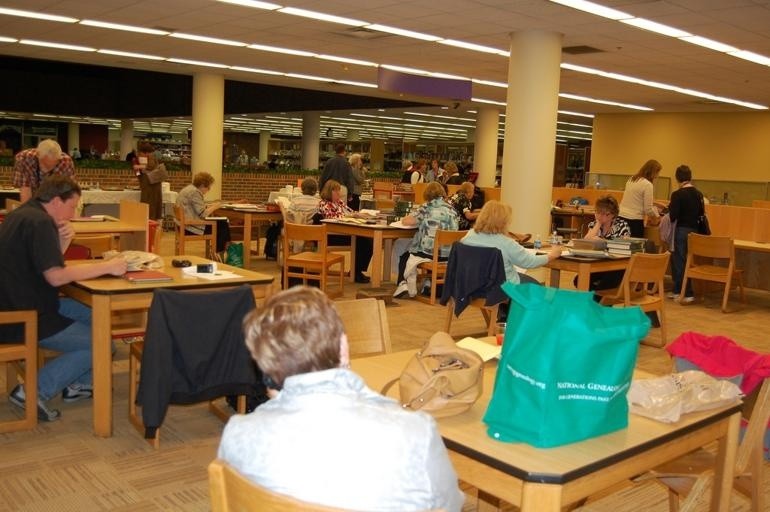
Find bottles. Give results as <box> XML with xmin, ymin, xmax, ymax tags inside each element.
<box><xmin>534</xmin><ymin>234</ymin><xmax>541</xmax><ymax>249</ymax></box>
<box><xmin>724</xmin><ymin>193</ymin><xmax>728</xmax><ymax>205</ymax></box>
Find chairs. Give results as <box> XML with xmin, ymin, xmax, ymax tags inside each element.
<box><xmin>69</xmin><ymin>233</ymin><xmax>119</xmax><ymax>260</ymax></box>
<box><xmin>643</xmin><ymin>331</ymin><xmax>770</xmax><ymax>511</ymax></box>
<box><xmin>599</xmin><ymin>252</ymin><xmax>671</xmax><ymax>346</ymax></box>
<box><xmin>209</xmin><ymin>459</ymin><xmax>342</xmax><ymax>511</ymax></box>
<box><xmin>418</xmin><ymin>229</ymin><xmax>470</xmax><ymax>306</ymax></box>
<box><xmin>334</xmin><ymin>298</ymin><xmax>391</xmax><ymax>358</ymax></box>
<box><xmin>117</xmin><ymin>200</ymin><xmax>149</xmax><ymax>253</ymax></box>
<box><xmin>373</xmin><ymin>182</ymin><xmax>396</xmax><ymax>213</ymax></box>
<box><xmin>678</xmin><ymin>233</ymin><xmax>746</xmax><ymax>313</ymax></box>
<box><xmin>1</xmin><ymin>310</ymin><xmax>37</xmax><ymax>433</ymax></box>
<box><xmin>283</xmin><ymin>222</ymin><xmax>345</xmax><ymax>298</ymax></box>
<box><xmin>173</xmin><ymin>205</ymin><xmax>216</xmax><ymax>263</ymax></box>
<box><xmin>129</xmin><ymin>284</ymin><xmax>253</xmax><ymax>450</ymax></box>
<box><xmin>444</xmin><ymin>241</ymin><xmax>511</xmax><ymax>337</ymax></box>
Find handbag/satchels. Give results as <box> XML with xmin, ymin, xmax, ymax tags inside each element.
<box><xmin>226</xmin><ymin>242</ymin><xmax>244</xmax><ymax>267</ymax></box>
<box><xmin>695</xmin><ymin>215</ymin><xmax>712</xmax><ymax>235</ymax></box>
<box><xmin>379</xmin><ymin>331</ymin><xmax>484</xmax><ymax>417</ymax></box>
<box><xmin>146</xmin><ymin>162</ymin><xmax>168</xmax><ymax>185</ymax></box>
<box><xmin>482</xmin><ymin>282</ymin><xmax>652</xmax><ymax>449</ymax></box>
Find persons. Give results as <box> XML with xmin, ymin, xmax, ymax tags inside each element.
<box><xmin>216</xmin><ymin>285</ymin><xmax>463</xmax><ymax>512</ymax></box>
<box><xmin>73</xmin><ymin>148</ymin><xmax>82</xmax><ymax>161</ymax></box>
<box><xmin>0</xmin><ymin>176</ymin><xmax>115</xmax><ymax>421</ymax></box>
<box><xmin>12</xmin><ymin>138</ymin><xmax>75</xmax><ymax>202</ymax></box>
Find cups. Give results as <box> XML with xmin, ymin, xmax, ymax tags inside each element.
<box><xmin>286</xmin><ymin>185</ymin><xmax>302</xmax><ymax>194</ymax></box>
<box><xmin>386</xmin><ymin>215</ymin><xmax>399</xmax><ymax>225</ymax></box>
<box><xmin>494</xmin><ymin>323</ymin><xmax>507</xmax><ymax>346</ymax></box>
<box><xmin>396</xmin><ymin>201</ymin><xmax>413</xmax><ymax>217</ymax></box>
<box><xmin>197</xmin><ymin>263</ymin><xmax>214</xmax><ymax>273</ymax></box>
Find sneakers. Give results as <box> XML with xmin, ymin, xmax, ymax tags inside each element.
<box><xmin>62</xmin><ymin>385</ymin><xmax>93</xmax><ymax>402</ymax></box>
<box><xmin>9</xmin><ymin>383</ymin><xmax>60</xmax><ymax>422</ymax></box>
<box><xmin>393</xmin><ymin>283</ymin><xmax>410</xmax><ymax>298</ymax></box>
<box><xmin>668</xmin><ymin>291</ymin><xmax>697</xmax><ymax>302</ymax></box>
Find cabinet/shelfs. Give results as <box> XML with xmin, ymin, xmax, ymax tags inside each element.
<box><xmin>383</xmin><ymin>139</ymin><xmax>405</xmax><ymax>169</ymax></box>
<box><xmin>137</xmin><ymin>140</ymin><xmax>191</xmax><ymax>158</ymax></box>
<box><xmin>267</xmin><ymin>138</ymin><xmax>384</xmax><ymax>171</ymax></box>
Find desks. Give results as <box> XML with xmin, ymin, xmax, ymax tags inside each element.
<box><xmin>61</xmin><ymin>255</ymin><xmax>274</xmax><ymax>438</ymax></box>
<box><xmin>80</xmin><ymin>185</ymin><xmax>179</xmax><ymax>205</ymax></box>
<box><xmin>474</xmin><ymin>208</ymin><xmax>481</xmax><ymax>226</ymax></box>
<box><xmin>70</xmin><ymin>219</ymin><xmax>145</xmax><ymax>235</ymax></box>
<box><xmin>270</xmin><ymin>190</ymin><xmax>415</xmax><ymax>206</ymax></box>
<box><xmin>735</xmin><ymin>239</ymin><xmax>769</xmax><ymax>252</ymax></box>
<box><xmin>1</xmin><ymin>186</ymin><xmax>21</xmax><ymax>209</ymax></box>
<box><xmin>208</xmin><ymin>201</ymin><xmax>283</xmax><ymax>272</ymax></box>
<box><xmin>351</xmin><ymin>333</ymin><xmax>744</xmax><ymax>510</ymax></box>
<box><xmin>552</xmin><ymin>204</ymin><xmax>621</xmax><ymax>238</ymax></box>
<box><xmin>524</xmin><ymin>244</ymin><xmax>632</xmax><ymax>293</ymax></box>
<box><xmin>321</xmin><ymin>216</ymin><xmax>420</xmax><ymax>288</ymax></box>
